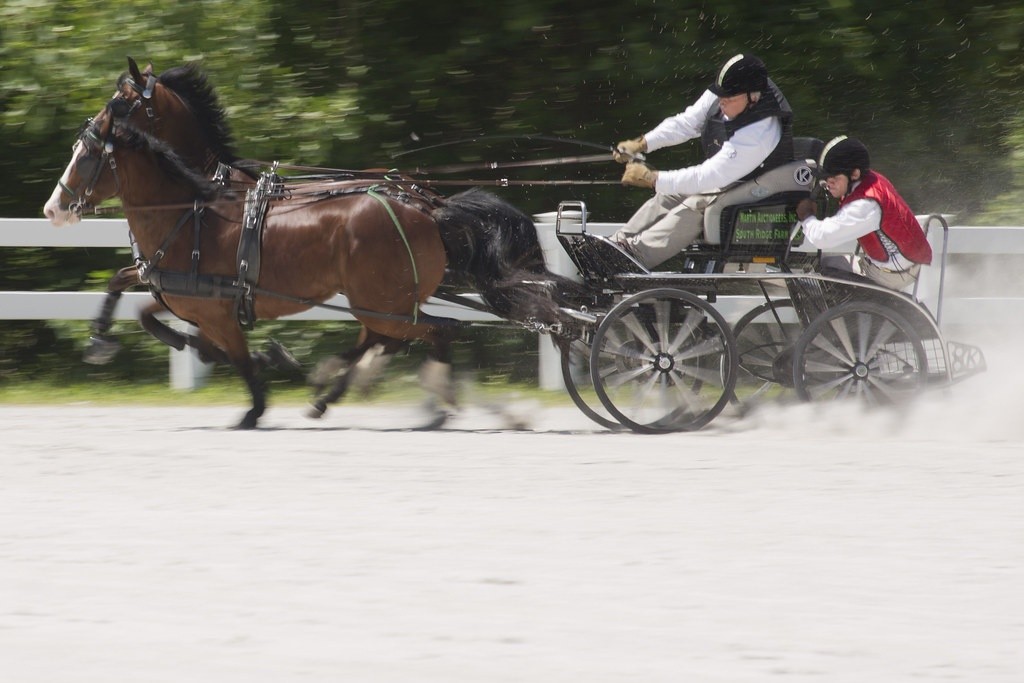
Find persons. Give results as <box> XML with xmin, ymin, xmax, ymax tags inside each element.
<box><xmin>606</xmin><ymin>54</ymin><xmax>794</xmax><ymax>272</ymax></box>
<box><xmin>797</xmin><ymin>136</ymin><xmax>932</xmax><ymax>291</ymax></box>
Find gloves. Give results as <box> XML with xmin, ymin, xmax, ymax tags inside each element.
<box><xmin>619</xmin><ymin>162</ymin><xmax>658</xmax><ymax>191</ymax></box>
<box><xmin>611</xmin><ymin>136</ymin><xmax>646</xmax><ymax>165</ymax></box>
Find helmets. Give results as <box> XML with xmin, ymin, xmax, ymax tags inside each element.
<box><xmin>708</xmin><ymin>52</ymin><xmax>771</xmax><ymax>97</ymax></box>
<box><xmin>809</xmin><ymin>135</ymin><xmax>870</xmax><ymax>176</ymax></box>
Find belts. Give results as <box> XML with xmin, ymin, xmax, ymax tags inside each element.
<box><xmin>870</xmin><ymin>261</ymin><xmax>918</xmax><ymax>275</ymax></box>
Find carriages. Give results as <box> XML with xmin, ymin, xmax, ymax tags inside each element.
<box><xmin>43</xmin><ymin>53</ymin><xmax>954</xmax><ymax>434</ymax></box>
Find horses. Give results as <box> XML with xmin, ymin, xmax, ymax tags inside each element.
<box><xmin>43</xmin><ymin>57</ymin><xmax>619</xmax><ymax>431</ymax></box>
